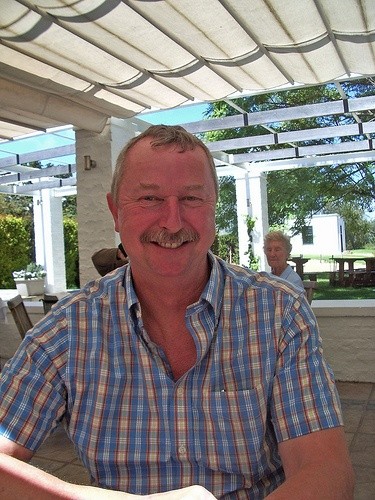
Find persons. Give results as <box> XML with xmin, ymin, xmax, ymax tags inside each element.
<box><xmin>91</xmin><ymin>243</ymin><xmax>128</xmax><ymax>277</ymax></box>
<box><xmin>263</xmin><ymin>230</ymin><xmax>305</xmax><ymax>290</ymax></box>
<box><xmin>0</xmin><ymin>124</ymin><xmax>356</xmax><ymax>500</ymax></box>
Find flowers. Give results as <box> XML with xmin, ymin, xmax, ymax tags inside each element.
<box><xmin>12</xmin><ymin>262</ymin><xmax>47</xmax><ymax>280</ymax></box>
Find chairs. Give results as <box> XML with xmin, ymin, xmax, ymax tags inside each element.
<box><xmin>7</xmin><ymin>295</ymin><xmax>34</xmax><ymax>341</ymax></box>
<box><xmin>302</xmin><ymin>281</ymin><xmax>316</xmax><ymax>307</ymax></box>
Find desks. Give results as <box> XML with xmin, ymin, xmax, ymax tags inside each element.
<box><xmin>287</xmin><ymin>257</ymin><xmax>311</xmax><ymax>281</ymax></box>
<box><xmin>330</xmin><ymin>258</ymin><xmax>362</xmax><ymax>286</ymax></box>
<box><xmin>362</xmin><ymin>257</ymin><xmax>375</xmax><ymax>272</ymax></box>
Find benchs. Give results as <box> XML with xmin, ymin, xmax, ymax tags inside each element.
<box><xmin>349</xmin><ymin>271</ymin><xmax>375</xmax><ymax>288</ymax></box>
<box><xmin>302</xmin><ymin>273</ymin><xmax>317</xmax><ymax>282</ymax></box>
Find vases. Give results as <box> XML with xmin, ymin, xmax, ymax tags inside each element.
<box><xmin>13</xmin><ymin>278</ymin><xmax>45</xmax><ymax>296</ymax></box>
